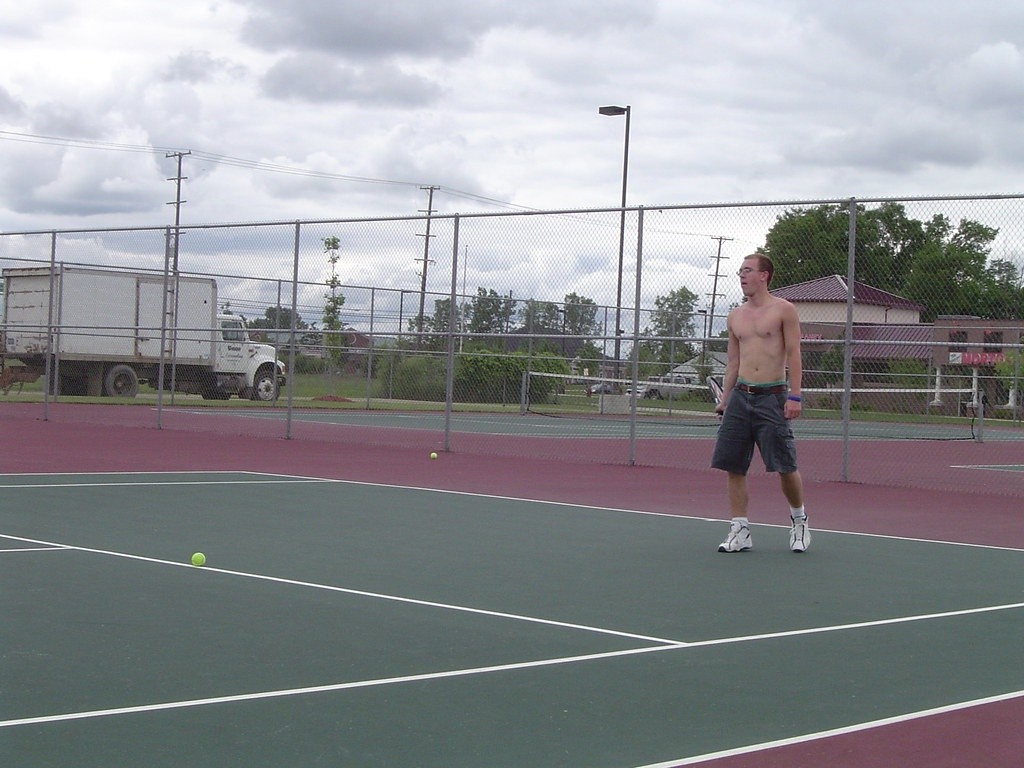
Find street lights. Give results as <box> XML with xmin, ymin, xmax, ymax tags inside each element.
<box><xmin>599</xmin><ymin>105</ymin><xmax>631</xmax><ymax>388</ymax></box>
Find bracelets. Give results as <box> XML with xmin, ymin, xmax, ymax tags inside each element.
<box><xmin>787</xmin><ymin>396</ymin><xmax>801</xmax><ymax>401</ymax></box>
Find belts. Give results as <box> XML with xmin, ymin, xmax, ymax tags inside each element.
<box><xmin>734</xmin><ymin>382</ymin><xmax>787</xmax><ymax>394</ymax></box>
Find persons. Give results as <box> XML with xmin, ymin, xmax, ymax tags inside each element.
<box><xmin>708</xmin><ymin>252</ymin><xmax>811</xmax><ymax>553</ymax></box>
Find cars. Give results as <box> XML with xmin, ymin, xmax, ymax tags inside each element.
<box><xmin>583</xmin><ymin>382</ymin><xmax>623</xmax><ymax>395</ymax></box>
<box><xmin>626</xmin><ymin>384</ymin><xmax>647</xmax><ymax>400</ymax></box>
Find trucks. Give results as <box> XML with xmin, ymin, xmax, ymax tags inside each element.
<box><xmin>2</xmin><ymin>264</ymin><xmax>288</xmax><ymax>402</ymax></box>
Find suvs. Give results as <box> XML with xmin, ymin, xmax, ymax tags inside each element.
<box><xmin>645</xmin><ymin>374</ymin><xmax>709</xmax><ymax>401</ymax></box>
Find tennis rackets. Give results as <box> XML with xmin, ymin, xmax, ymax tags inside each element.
<box><xmin>705</xmin><ymin>374</ymin><xmax>726</xmax><ymax>419</ymax></box>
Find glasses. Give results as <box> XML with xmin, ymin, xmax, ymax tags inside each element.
<box><xmin>736</xmin><ymin>268</ymin><xmax>765</xmax><ymax>275</ymax></box>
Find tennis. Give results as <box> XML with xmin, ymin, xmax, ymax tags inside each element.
<box><xmin>191</xmin><ymin>552</ymin><xmax>207</xmax><ymax>567</ymax></box>
<box><xmin>430</xmin><ymin>452</ymin><xmax>437</xmax><ymax>460</ymax></box>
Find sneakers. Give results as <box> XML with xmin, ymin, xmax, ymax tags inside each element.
<box><xmin>718</xmin><ymin>521</ymin><xmax>752</xmax><ymax>552</ymax></box>
<box><xmin>790</xmin><ymin>513</ymin><xmax>811</xmax><ymax>552</ymax></box>
